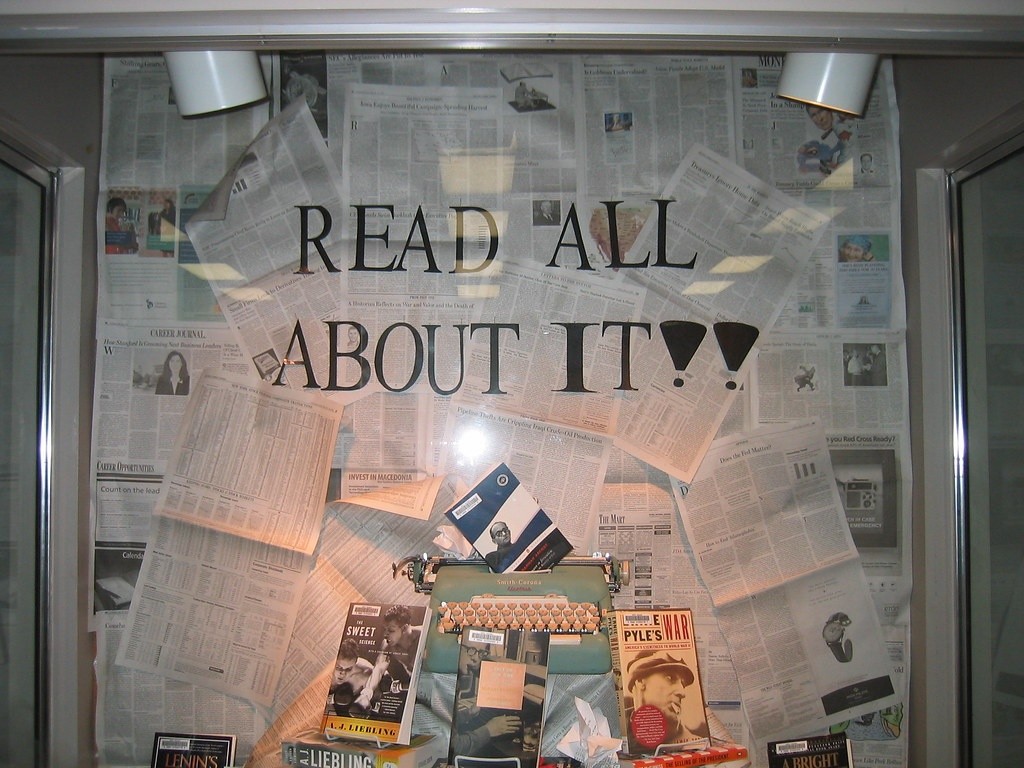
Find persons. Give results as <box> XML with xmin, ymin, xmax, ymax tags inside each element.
<box><xmin>839</xmin><ymin>235</ymin><xmax>876</xmax><ymax>262</ymax></box>
<box><xmin>806</xmin><ymin>103</ymin><xmax>833</xmax><ymax>130</ymax></box>
<box><xmin>155</xmin><ymin>351</ymin><xmax>190</xmax><ymax>394</ymax></box>
<box><xmin>861</xmin><ymin>154</ymin><xmax>873</xmax><ymax>173</ymax></box>
<box><xmin>515</xmin><ymin>81</ymin><xmax>539</xmax><ymax>109</ymax></box>
<box><xmin>105</xmin><ymin>197</ymin><xmax>138</xmax><ymax>254</ymax></box>
<box><xmin>794</xmin><ymin>365</ymin><xmax>815</xmax><ymax>392</ymax></box>
<box><xmin>533</xmin><ymin>201</ymin><xmax>560</xmax><ymax>224</ymax></box>
<box><xmin>448</xmin><ymin>628</ymin><xmax>522</xmax><ymax>762</ymax></box>
<box><xmin>148</xmin><ymin>198</ymin><xmax>176</xmax><ymax>257</ymax></box>
<box><xmin>624</xmin><ymin>650</ymin><xmax>707</xmax><ymax>754</ymax></box>
<box><xmin>326</xmin><ymin>605</ymin><xmax>423</xmax><ymax>719</ymax></box>
<box><xmin>843</xmin><ymin>344</ymin><xmax>887</xmax><ymax>386</ymax></box>
<box><xmin>613</xmin><ymin>668</ymin><xmax>623</xmax><ymax>691</ymax></box>
<box><xmin>485</xmin><ymin>521</ymin><xmax>513</xmax><ymax>572</ymax></box>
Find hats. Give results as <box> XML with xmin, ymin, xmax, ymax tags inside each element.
<box><xmin>628</xmin><ymin>649</ymin><xmax>694</xmax><ymax>692</ymax></box>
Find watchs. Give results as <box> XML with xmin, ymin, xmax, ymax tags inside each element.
<box><xmin>821</xmin><ymin>611</ymin><xmax>853</xmax><ymax>662</ymax></box>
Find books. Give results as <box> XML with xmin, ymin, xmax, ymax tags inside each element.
<box><xmin>607</xmin><ymin>608</ymin><xmax>711</xmax><ymax>756</ymax></box>
<box><xmin>448</xmin><ymin>625</ymin><xmax>551</xmax><ymax>768</ymax></box>
<box><xmin>443</xmin><ymin>462</ymin><xmax>574</xmax><ymax>573</ymax></box>
<box><xmin>151</xmin><ymin>732</ymin><xmax>237</xmax><ymax>768</ymax></box>
<box><xmin>281</xmin><ymin>728</ymin><xmax>437</xmax><ymax>768</ymax></box>
<box><xmin>319</xmin><ymin>602</ymin><xmax>432</xmax><ymax>745</ymax></box>
<box><xmin>767</xmin><ymin>731</ymin><xmax>856</xmax><ymax>768</ymax></box>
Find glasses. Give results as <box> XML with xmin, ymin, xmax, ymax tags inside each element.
<box><xmin>462</xmin><ymin>645</ymin><xmax>490</xmax><ymax>659</ymax></box>
<box><xmin>666</xmin><ymin>652</ymin><xmax>689</xmax><ymax>666</ymax></box>
<box><xmin>494</xmin><ymin>528</ymin><xmax>508</xmax><ymax>537</ymax></box>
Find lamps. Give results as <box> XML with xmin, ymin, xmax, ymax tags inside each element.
<box><xmin>776</xmin><ymin>51</ymin><xmax>882</xmax><ymax>116</ymax></box>
<box><xmin>160</xmin><ymin>52</ymin><xmax>270</xmax><ymax>122</ymax></box>
